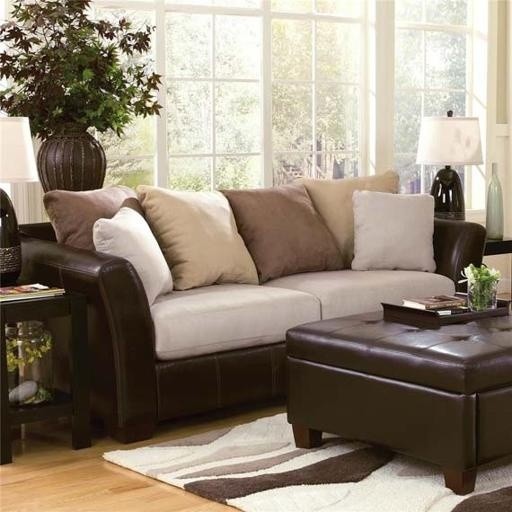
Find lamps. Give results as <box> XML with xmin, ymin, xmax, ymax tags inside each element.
<box><xmin>0</xmin><ymin>118</ymin><xmax>40</xmax><ymax>287</ymax></box>
<box><xmin>415</xmin><ymin>111</ymin><xmax>483</xmax><ymax>220</ymax></box>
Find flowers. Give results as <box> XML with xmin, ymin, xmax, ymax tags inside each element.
<box><xmin>457</xmin><ymin>264</ymin><xmax>501</xmax><ymax>310</ymax></box>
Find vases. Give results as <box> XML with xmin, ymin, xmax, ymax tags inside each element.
<box><xmin>467</xmin><ymin>280</ymin><xmax>497</xmax><ymax>312</ymax></box>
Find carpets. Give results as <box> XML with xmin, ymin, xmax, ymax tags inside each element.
<box><xmin>101</xmin><ymin>410</ymin><xmax>512</xmax><ymax>511</ymax></box>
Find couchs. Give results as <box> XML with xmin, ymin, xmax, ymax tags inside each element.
<box><xmin>16</xmin><ymin>218</ymin><xmax>487</xmax><ymax>444</ymax></box>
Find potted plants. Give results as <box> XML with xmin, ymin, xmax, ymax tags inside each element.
<box><xmin>0</xmin><ymin>0</ymin><xmax>162</xmax><ymax>192</ymax></box>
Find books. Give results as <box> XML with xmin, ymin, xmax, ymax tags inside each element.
<box><xmin>0</xmin><ymin>282</ymin><xmax>67</xmax><ymax>303</ymax></box>
<box><xmin>401</xmin><ymin>295</ymin><xmax>470</xmax><ymax>316</ymax></box>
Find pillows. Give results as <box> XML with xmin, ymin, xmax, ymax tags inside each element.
<box><xmin>136</xmin><ymin>184</ymin><xmax>259</xmax><ymax>290</ymax></box>
<box><xmin>219</xmin><ymin>182</ymin><xmax>347</xmax><ymax>284</ymax></box>
<box><xmin>92</xmin><ymin>199</ymin><xmax>173</xmax><ymax>308</ymax></box>
<box><xmin>43</xmin><ymin>184</ymin><xmax>136</xmax><ymax>298</ymax></box>
<box><xmin>350</xmin><ymin>190</ymin><xmax>437</xmax><ymax>273</ymax></box>
<box><xmin>302</xmin><ymin>171</ymin><xmax>399</xmax><ymax>268</ymax></box>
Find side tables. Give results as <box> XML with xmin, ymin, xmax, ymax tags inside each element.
<box><xmin>0</xmin><ymin>281</ymin><xmax>91</xmax><ymax>464</ymax></box>
